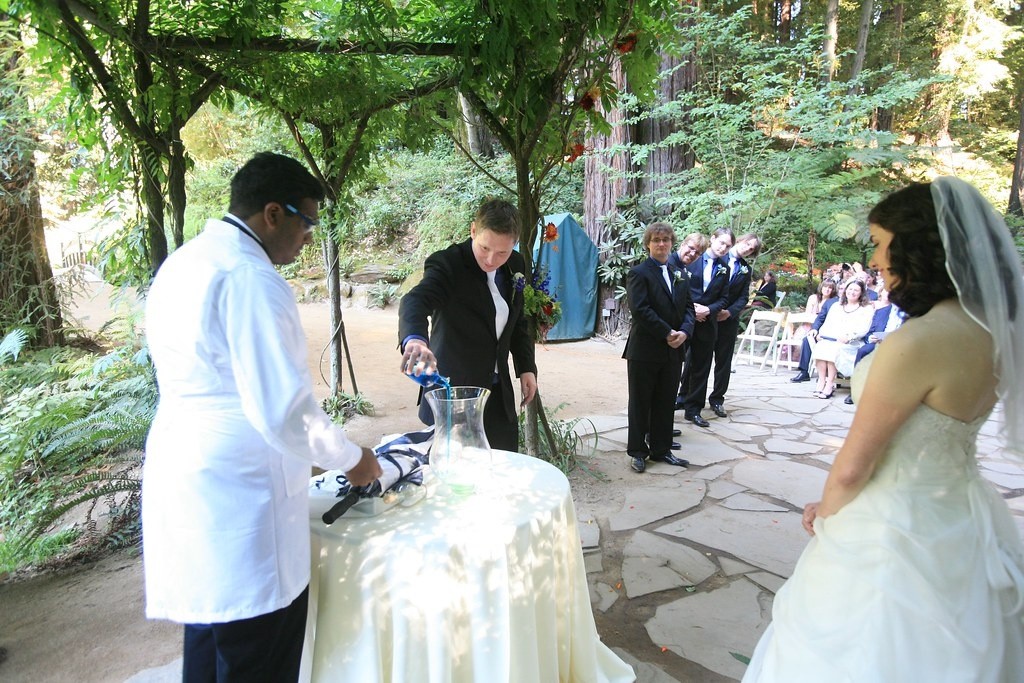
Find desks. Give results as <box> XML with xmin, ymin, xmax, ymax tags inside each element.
<box><xmin>296</xmin><ymin>448</ymin><xmax>637</xmax><ymax>683</ymax></box>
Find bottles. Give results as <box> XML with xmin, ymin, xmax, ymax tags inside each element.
<box><xmin>400</xmin><ymin>356</ymin><xmax>451</xmax><ymax>389</ymax></box>
<box><xmin>424</xmin><ymin>385</ymin><xmax>493</xmax><ymax>489</ymax></box>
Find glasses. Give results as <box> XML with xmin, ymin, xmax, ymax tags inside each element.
<box><xmin>648</xmin><ymin>237</ymin><xmax>673</xmax><ymax>243</ymax></box>
<box><xmin>263</xmin><ymin>199</ymin><xmax>320</xmax><ymax>233</ymax></box>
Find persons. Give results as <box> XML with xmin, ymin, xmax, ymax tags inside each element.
<box><xmin>142</xmin><ymin>153</ymin><xmax>383</xmax><ymax>683</ymax></box>
<box><xmin>747</xmin><ymin>268</ymin><xmax>778</xmax><ymax>308</ymax></box>
<box><xmin>397</xmin><ymin>197</ymin><xmax>539</xmax><ymax>454</ymax></box>
<box><xmin>685</xmin><ymin>227</ymin><xmax>736</xmax><ymax>427</ymax></box>
<box><xmin>824</xmin><ymin>261</ymin><xmax>892</xmax><ymax>301</ymax></box>
<box><xmin>790</xmin><ymin>274</ymin><xmax>871</xmax><ymax>383</ymax></box>
<box><xmin>728</xmin><ymin>234</ymin><xmax>763</xmax><ymax>349</ymax></box>
<box><xmin>623</xmin><ymin>222</ymin><xmax>697</xmax><ymax>474</ymax></box>
<box><xmin>796</xmin><ymin>278</ymin><xmax>838</xmax><ymax>356</ymax></box>
<box><xmin>741</xmin><ymin>174</ymin><xmax>1024</xmax><ymax>683</ymax></box>
<box><xmin>812</xmin><ymin>280</ymin><xmax>875</xmax><ymax>399</ymax></box>
<box><xmin>670</xmin><ymin>230</ymin><xmax>709</xmax><ymax>450</ymax></box>
<box><xmin>843</xmin><ymin>299</ymin><xmax>910</xmax><ymax>405</ymax></box>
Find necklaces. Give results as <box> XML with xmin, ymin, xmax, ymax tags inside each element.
<box><xmin>841</xmin><ymin>302</ymin><xmax>861</xmax><ymax>314</ymax></box>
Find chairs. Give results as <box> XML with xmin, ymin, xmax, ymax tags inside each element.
<box><xmin>730</xmin><ymin>310</ymin><xmax>850</xmax><ymax>392</ymax></box>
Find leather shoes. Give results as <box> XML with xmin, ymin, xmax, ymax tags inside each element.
<box><xmin>631</xmin><ymin>457</ymin><xmax>645</xmax><ymax>473</ymax></box>
<box><xmin>789</xmin><ymin>372</ymin><xmax>810</xmax><ymax>383</ymax></box>
<box><xmin>649</xmin><ymin>452</ymin><xmax>690</xmax><ymax>467</ymax></box>
<box><xmin>684</xmin><ymin>414</ymin><xmax>710</xmax><ymax>427</ymax></box>
<box><xmin>710</xmin><ymin>404</ymin><xmax>728</xmax><ymax>417</ymax></box>
<box><xmin>675</xmin><ymin>402</ymin><xmax>684</xmax><ymax>410</ymax></box>
<box><xmin>671</xmin><ymin>442</ymin><xmax>681</xmax><ymax>450</ymax></box>
<box><xmin>844</xmin><ymin>395</ymin><xmax>853</xmax><ymax>404</ymax></box>
<box><xmin>673</xmin><ymin>429</ymin><xmax>682</xmax><ymax>436</ymax></box>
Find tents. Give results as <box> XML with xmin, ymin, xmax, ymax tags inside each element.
<box><xmin>513</xmin><ymin>210</ymin><xmax>607</xmax><ymax>344</ymax></box>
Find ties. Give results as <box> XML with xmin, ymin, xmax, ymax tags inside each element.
<box><xmin>660</xmin><ymin>265</ymin><xmax>672</xmax><ymax>294</ymax></box>
<box><xmin>486</xmin><ymin>270</ymin><xmax>509</xmax><ymax>375</ymax></box>
<box><xmin>728</xmin><ymin>257</ymin><xmax>737</xmax><ymax>280</ymax></box>
<box><xmin>703</xmin><ymin>259</ymin><xmax>713</xmax><ymax>292</ymax></box>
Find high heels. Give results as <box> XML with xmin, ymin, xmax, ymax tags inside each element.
<box><xmin>818</xmin><ymin>384</ymin><xmax>834</xmax><ymax>399</ymax></box>
<box><xmin>812</xmin><ymin>381</ymin><xmax>826</xmax><ymax>398</ymax></box>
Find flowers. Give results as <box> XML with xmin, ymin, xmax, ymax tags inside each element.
<box><xmin>511</xmin><ymin>272</ymin><xmax>526</xmax><ymax>302</ymax></box>
